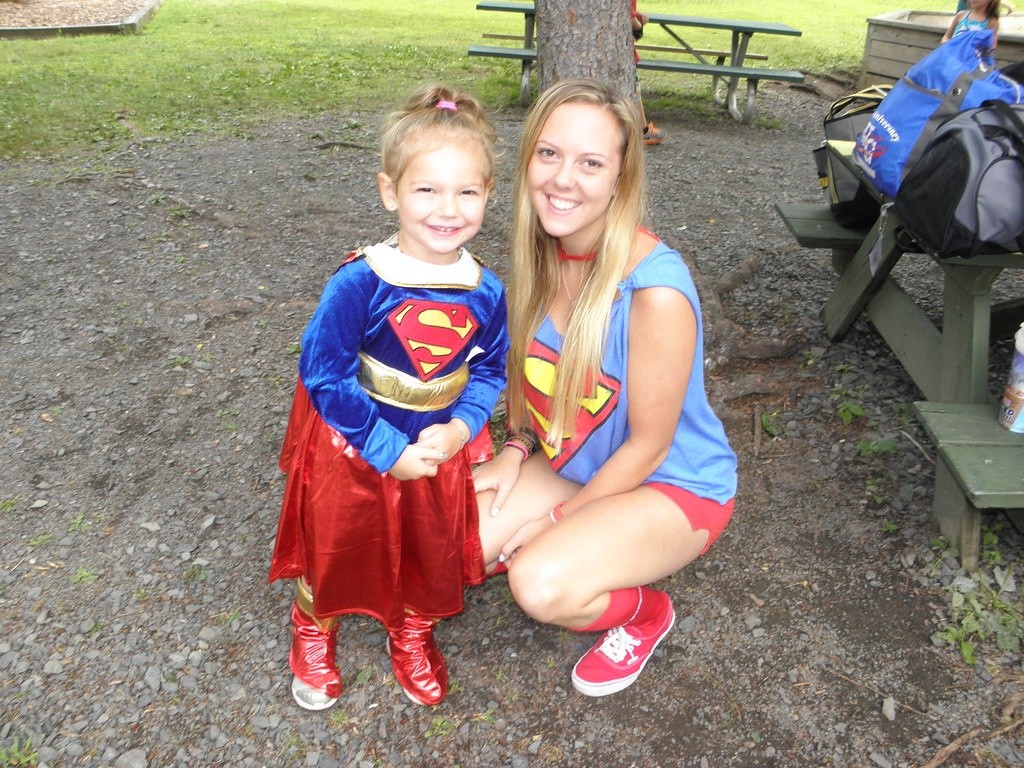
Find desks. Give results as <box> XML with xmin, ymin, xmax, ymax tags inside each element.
<box><xmin>477</xmin><ymin>2</ymin><xmax>803</xmax><ymax>122</ymax></box>
<box><xmin>819</xmin><ymin>139</ymin><xmax>1024</xmax><ymax>570</ymax></box>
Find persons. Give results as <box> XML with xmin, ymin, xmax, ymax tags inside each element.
<box><xmin>940</xmin><ymin>0</ymin><xmax>1001</xmax><ymax>49</ymax></box>
<box><xmin>471</xmin><ymin>78</ymin><xmax>740</xmax><ymax>698</ymax></box>
<box><xmin>266</xmin><ymin>84</ymin><xmax>511</xmax><ymax>714</ymax></box>
<box><xmin>629</xmin><ymin>0</ymin><xmax>668</xmax><ymax>146</ymax></box>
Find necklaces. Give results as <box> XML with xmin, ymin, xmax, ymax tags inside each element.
<box><xmin>560</xmin><ymin>249</ymin><xmax>596</xmax><ymax>264</ymax></box>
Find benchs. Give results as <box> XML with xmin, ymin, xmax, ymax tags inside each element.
<box><xmin>468</xmin><ymin>44</ymin><xmax>805</xmax><ymax>125</ymax></box>
<box><xmin>774</xmin><ymin>203</ymin><xmax>926</xmax><ymax>273</ymax></box>
<box><xmin>911</xmin><ymin>401</ymin><xmax>1024</xmax><ymax>511</ymax></box>
<box><xmin>482</xmin><ymin>33</ymin><xmax>768</xmax><ymax>110</ymax></box>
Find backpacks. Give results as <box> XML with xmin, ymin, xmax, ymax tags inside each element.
<box><xmin>851</xmin><ymin>30</ymin><xmax>1024</xmax><ymax>200</ymax></box>
<box><xmin>813</xmin><ymin>84</ymin><xmax>893</xmax><ymax>235</ymax></box>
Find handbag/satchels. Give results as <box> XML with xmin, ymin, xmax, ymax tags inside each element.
<box><xmin>893</xmin><ymin>100</ymin><xmax>1024</xmax><ymax>259</ymax></box>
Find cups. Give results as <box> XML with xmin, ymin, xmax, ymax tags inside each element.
<box><xmin>997</xmin><ymin>321</ymin><xmax>1024</xmax><ymax>433</ymax></box>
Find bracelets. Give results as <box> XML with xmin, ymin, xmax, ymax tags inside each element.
<box><xmin>550</xmin><ymin>503</ymin><xmax>564</xmax><ymax>523</ymax></box>
<box><xmin>503</xmin><ymin>426</ymin><xmax>542</xmax><ymax>462</ymax></box>
<box><xmin>448</xmin><ymin>421</ymin><xmax>466</xmax><ymax>451</ymax></box>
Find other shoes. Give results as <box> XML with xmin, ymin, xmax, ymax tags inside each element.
<box><xmin>289</xmin><ymin>600</ymin><xmax>342</xmax><ymax>698</ymax></box>
<box><xmin>571</xmin><ymin>593</ymin><xmax>675</xmax><ymax>697</ymax></box>
<box><xmin>387</xmin><ymin>613</ymin><xmax>448</xmax><ymax>706</ymax></box>
